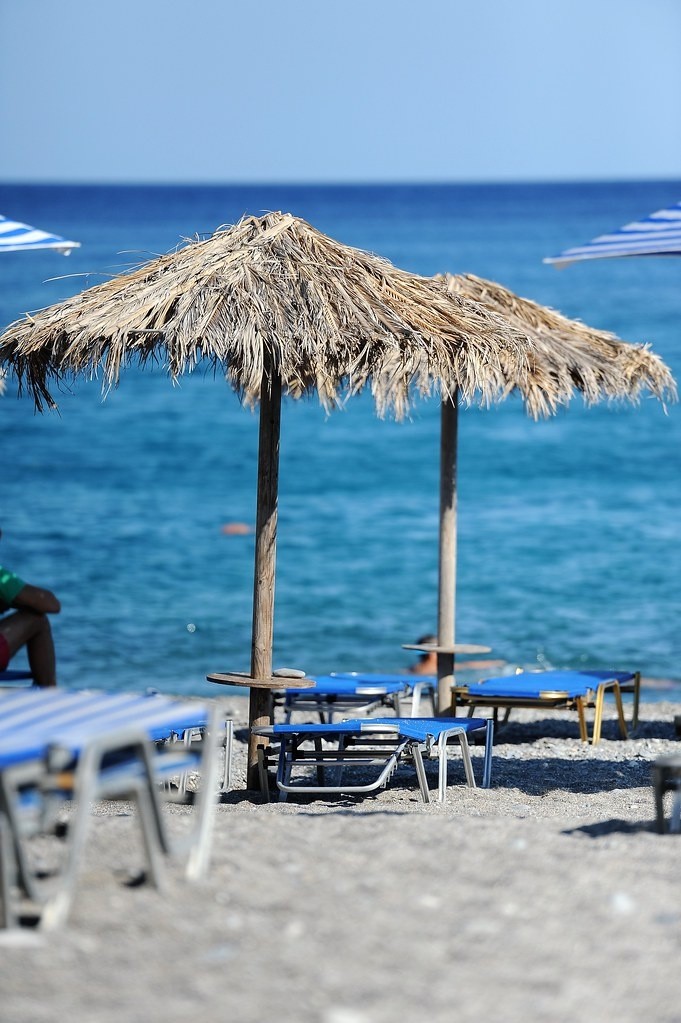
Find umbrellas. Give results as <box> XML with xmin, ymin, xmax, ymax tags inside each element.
<box><xmin>539</xmin><ymin>193</ymin><xmax>681</xmax><ymax>266</ymax></box>
<box><xmin>0</xmin><ymin>212</ymin><xmax>81</xmax><ymax>259</ymax></box>
<box><xmin>0</xmin><ymin>209</ymin><xmax>680</xmax><ymax>798</ymax></box>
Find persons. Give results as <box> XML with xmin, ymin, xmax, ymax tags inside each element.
<box><xmin>0</xmin><ymin>528</ymin><xmax>59</xmax><ymax>689</ymax></box>
<box><xmin>411</xmin><ymin>634</ymin><xmax>506</xmax><ymax>675</ymax></box>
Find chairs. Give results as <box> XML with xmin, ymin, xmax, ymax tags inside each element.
<box><xmin>0</xmin><ymin>671</ymin><xmax>681</xmax><ymax>931</ymax></box>
